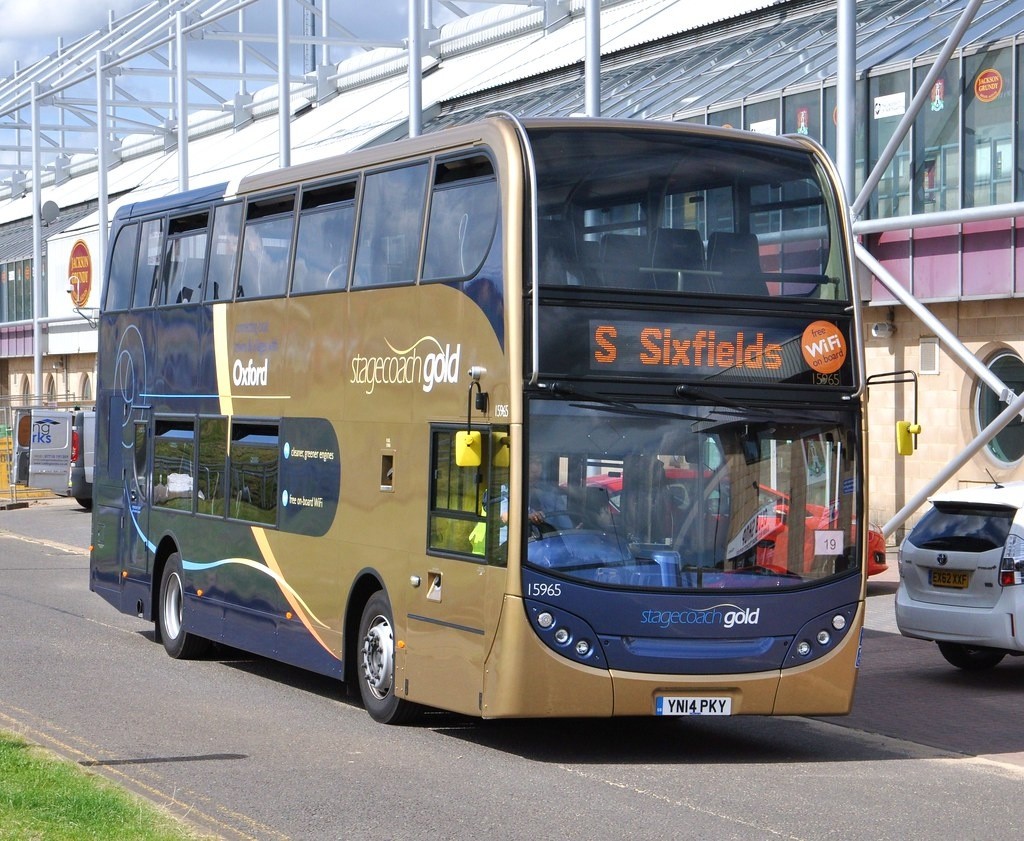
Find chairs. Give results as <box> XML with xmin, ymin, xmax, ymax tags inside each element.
<box><xmin>538</xmin><ymin>219</ymin><xmax>770</xmax><ymax>295</ymax></box>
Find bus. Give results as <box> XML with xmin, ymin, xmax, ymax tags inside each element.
<box><xmin>81</xmin><ymin>110</ymin><xmax>923</xmax><ymax>724</ymax></box>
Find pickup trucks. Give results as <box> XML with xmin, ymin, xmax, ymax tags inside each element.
<box><xmin>8</xmin><ymin>406</ymin><xmax>94</xmax><ymax>510</ymax></box>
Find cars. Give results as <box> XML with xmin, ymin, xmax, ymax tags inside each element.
<box><xmin>894</xmin><ymin>481</ymin><xmax>1024</xmax><ymax>671</ymax></box>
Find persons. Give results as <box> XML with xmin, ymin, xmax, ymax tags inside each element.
<box><xmin>483</xmin><ymin>455</ymin><xmax>576</xmax><ymax>549</ymax></box>
